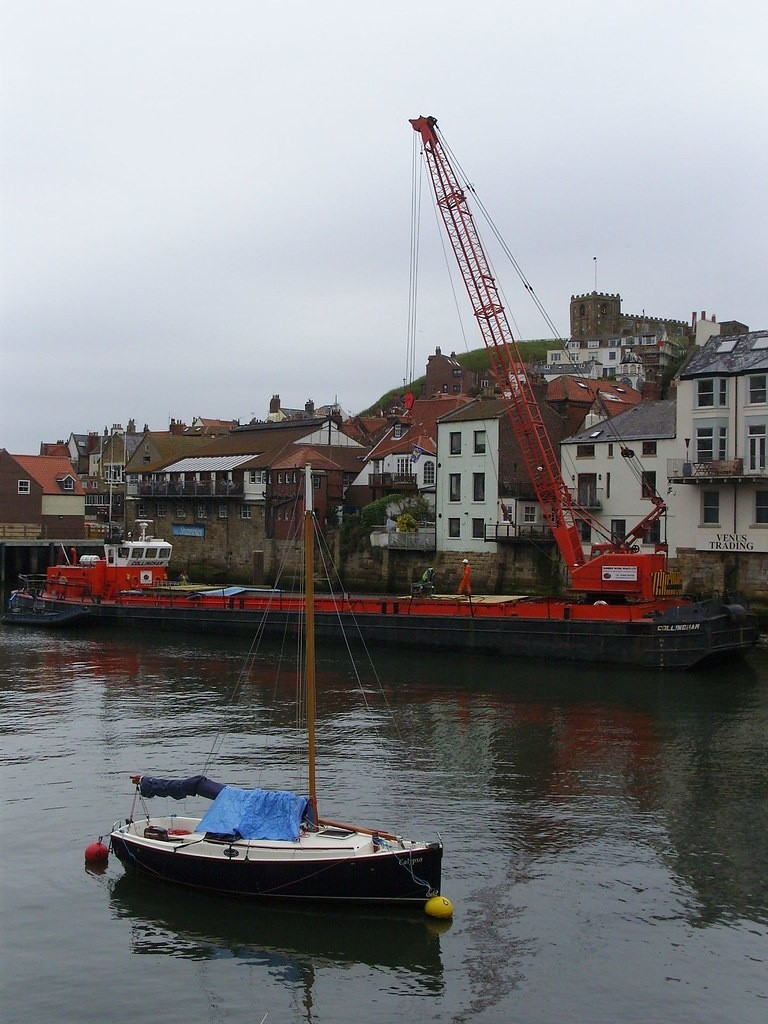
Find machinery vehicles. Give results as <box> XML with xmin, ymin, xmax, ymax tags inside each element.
<box><xmin>409</xmin><ymin>113</ymin><xmax>670</xmax><ymax>609</ymax></box>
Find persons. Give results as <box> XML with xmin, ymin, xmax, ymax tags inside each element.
<box><xmin>178</xmin><ymin>569</ymin><xmax>195</xmax><ymax>592</ymax></box>
<box><xmin>140</xmin><ymin>475</ymin><xmax>244</xmax><ymax>495</ymax></box>
<box><xmin>420</xmin><ymin>563</ymin><xmax>440</xmax><ymax>596</ymax></box>
<box><xmin>457</xmin><ymin>558</ymin><xmax>472</xmax><ymax>596</ymax></box>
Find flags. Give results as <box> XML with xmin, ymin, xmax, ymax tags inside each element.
<box><xmin>411</xmin><ymin>445</ymin><xmax>424</xmax><ymax>463</ymax></box>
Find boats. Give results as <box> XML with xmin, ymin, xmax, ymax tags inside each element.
<box><xmin>8</xmin><ymin>516</ymin><xmax>762</xmax><ymax>677</ymax></box>
<box><xmin>1</xmin><ymin>586</ymin><xmax>92</xmax><ymax>630</ymax></box>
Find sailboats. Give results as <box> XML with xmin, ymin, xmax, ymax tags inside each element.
<box><xmin>110</xmin><ymin>458</ymin><xmax>444</xmax><ymax>908</ymax></box>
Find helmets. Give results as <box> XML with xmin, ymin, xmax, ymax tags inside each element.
<box><xmin>462</xmin><ymin>559</ymin><xmax>468</xmax><ymax>563</ymax></box>
